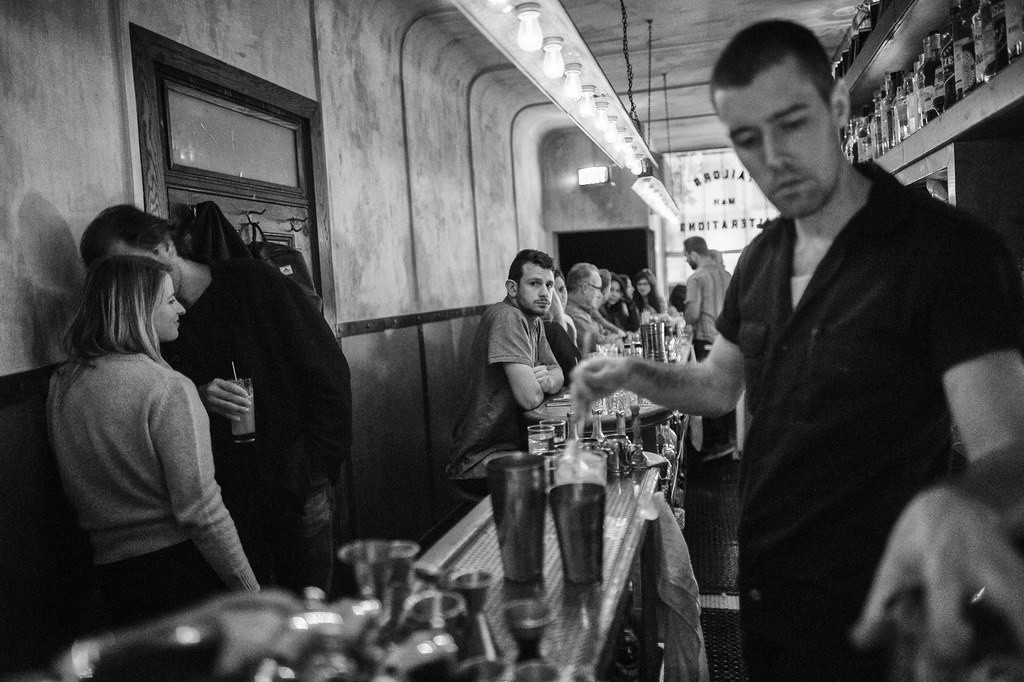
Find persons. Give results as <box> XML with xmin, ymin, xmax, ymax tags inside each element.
<box><xmin>80</xmin><ymin>201</ymin><xmax>357</xmax><ymax>604</ymax></box>
<box><xmin>569</xmin><ymin>19</ymin><xmax>1023</xmax><ymax>666</ymax></box>
<box><xmin>45</xmin><ymin>253</ymin><xmax>263</xmax><ymax>630</ymax></box>
<box><xmin>441</xmin><ymin>233</ymin><xmax>739</xmax><ymax>500</ymax></box>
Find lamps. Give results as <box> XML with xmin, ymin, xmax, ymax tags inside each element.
<box><xmin>629</xmin><ymin>17</ymin><xmax>681</xmax><ymax>225</ymax></box>
<box><xmin>577</xmin><ymin>164</ymin><xmax>613</xmax><ymax>189</ymax></box>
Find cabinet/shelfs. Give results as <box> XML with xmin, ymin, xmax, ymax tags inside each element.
<box><xmin>833</xmin><ymin>0</ymin><xmax>1024</xmax><ymax>214</ymax></box>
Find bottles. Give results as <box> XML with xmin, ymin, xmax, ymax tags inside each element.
<box><xmin>567</xmin><ymin>402</ymin><xmax>646</xmax><ymax>450</ymax></box>
<box><xmin>808</xmin><ymin>0</ymin><xmax>1024</xmax><ymax>161</ymax></box>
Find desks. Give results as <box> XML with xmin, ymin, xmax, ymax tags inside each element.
<box><xmin>412</xmin><ymin>450</ymin><xmax>675</xmax><ymax>681</ymax></box>
<box><xmin>521</xmin><ymin>323</ymin><xmax>699</xmax><ymax>534</ymax></box>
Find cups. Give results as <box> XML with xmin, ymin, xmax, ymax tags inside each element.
<box><xmin>228</xmin><ymin>376</ymin><xmax>255</xmax><ymax>443</ymax></box>
<box><xmin>577</xmin><ymin>310</ymin><xmax>700</xmax><ymax>409</ymax></box>
<box><xmin>321</xmin><ymin>416</ymin><xmax>648</xmax><ymax>678</ymax></box>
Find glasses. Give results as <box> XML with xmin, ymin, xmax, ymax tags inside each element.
<box><xmin>582</xmin><ymin>282</ymin><xmax>603</xmax><ymax>293</ymax></box>
